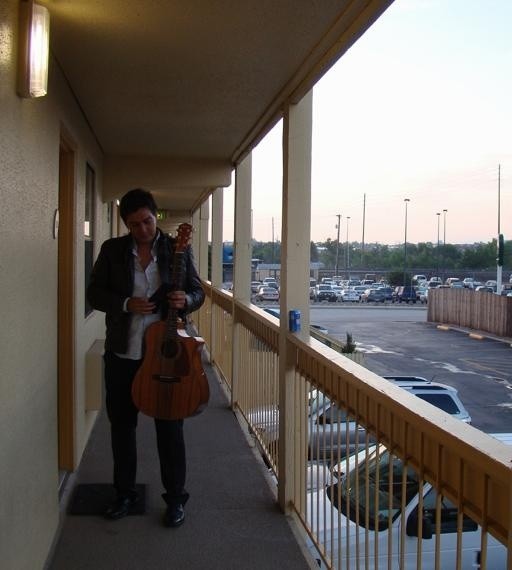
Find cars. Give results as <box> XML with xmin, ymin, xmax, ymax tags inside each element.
<box><xmin>250</xmin><ymin>274</ymin><xmax>512</xmax><ymax>303</ymax></box>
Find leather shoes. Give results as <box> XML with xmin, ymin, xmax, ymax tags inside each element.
<box><xmin>162</xmin><ymin>503</ymin><xmax>185</xmax><ymax>527</ymax></box>
<box><xmin>105</xmin><ymin>497</ymin><xmax>149</xmax><ymax>521</ymax></box>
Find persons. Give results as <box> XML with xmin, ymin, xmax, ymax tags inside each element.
<box><xmin>87</xmin><ymin>187</ymin><xmax>205</xmax><ymax>528</ymax></box>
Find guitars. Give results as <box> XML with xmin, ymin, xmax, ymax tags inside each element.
<box><xmin>132</xmin><ymin>223</ymin><xmax>210</xmax><ymax>420</ymax></box>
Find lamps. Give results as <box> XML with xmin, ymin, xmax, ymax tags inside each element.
<box><xmin>17</xmin><ymin>0</ymin><xmax>50</xmax><ymax>98</ymax></box>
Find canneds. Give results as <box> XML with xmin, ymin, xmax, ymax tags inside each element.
<box><xmin>288</xmin><ymin>309</ymin><xmax>301</xmax><ymax>332</ymax></box>
